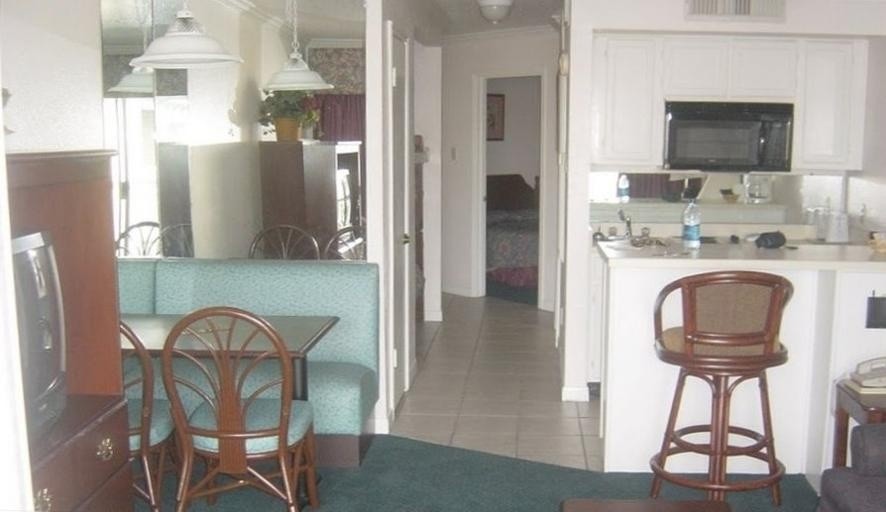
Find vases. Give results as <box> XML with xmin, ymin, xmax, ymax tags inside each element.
<box><xmin>272</xmin><ymin>114</ymin><xmax>303</xmax><ymax>144</ymax></box>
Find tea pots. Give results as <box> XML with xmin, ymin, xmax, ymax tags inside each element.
<box><xmin>745</xmin><ymin>182</ymin><xmax>773</xmax><ymax>205</ymax></box>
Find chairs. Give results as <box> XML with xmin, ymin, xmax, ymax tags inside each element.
<box><xmin>322</xmin><ymin>226</ymin><xmax>367</xmax><ymax>261</ymax></box>
<box><xmin>115</xmin><ymin>221</ymin><xmax>159</xmax><ymax>257</ymax></box>
<box><xmin>119</xmin><ymin>321</ymin><xmax>189</xmax><ymax>512</ymax></box>
<box><xmin>161</xmin><ymin>306</ymin><xmax>320</xmax><ymax>511</ymax></box>
<box><xmin>649</xmin><ymin>270</ymin><xmax>794</xmax><ymax>510</ymax></box>
<box><xmin>148</xmin><ymin>223</ymin><xmax>190</xmax><ymax>258</ymax></box>
<box><xmin>247</xmin><ymin>223</ymin><xmax>321</xmax><ymax>260</ymax></box>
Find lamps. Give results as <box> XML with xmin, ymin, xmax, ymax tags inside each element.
<box><xmin>475</xmin><ymin>0</ymin><xmax>515</xmax><ymax>24</ymax></box>
<box><xmin>128</xmin><ymin>0</ymin><xmax>245</xmax><ymax>68</ymax></box>
<box><xmin>263</xmin><ymin>1</ymin><xmax>335</xmax><ymax>92</ymax></box>
<box><xmin>107</xmin><ymin>1</ymin><xmax>155</xmax><ymax>93</ymax></box>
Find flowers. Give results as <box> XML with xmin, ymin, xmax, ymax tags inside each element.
<box><xmin>255</xmin><ymin>88</ymin><xmax>326</xmax><ymax>129</ymax></box>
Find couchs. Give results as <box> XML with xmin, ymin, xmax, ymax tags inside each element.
<box><xmin>817</xmin><ymin>423</ymin><xmax>886</xmax><ymax>511</ymax></box>
<box><xmin>118</xmin><ymin>257</ymin><xmax>379</xmax><ymax>469</ymax></box>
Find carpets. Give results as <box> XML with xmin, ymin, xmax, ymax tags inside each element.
<box><xmin>135</xmin><ymin>433</ymin><xmax>821</xmax><ymax>511</ymax></box>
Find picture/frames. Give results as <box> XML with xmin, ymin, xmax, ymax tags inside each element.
<box><xmin>486</xmin><ymin>93</ymin><xmax>505</xmax><ymax>141</ymax></box>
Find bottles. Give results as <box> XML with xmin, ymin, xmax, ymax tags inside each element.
<box><xmin>682</xmin><ymin>198</ymin><xmax>702</xmax><ymax>248</ymax></box>
<box><xmin>617</xmin><ymin>173</ymin><xmax>631</xmax><ymax>202</ymax></box>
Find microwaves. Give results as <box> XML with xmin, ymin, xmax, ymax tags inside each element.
<box><xmin>664</xmin><ymin>102</ymin><xmax>792</xmax><ymax>178</ymax></box>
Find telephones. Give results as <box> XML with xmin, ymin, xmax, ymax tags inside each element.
<box><xmin>849</xmin><ymin>356</ymin><xmax>886</xmax><ymax>387</ymax></box>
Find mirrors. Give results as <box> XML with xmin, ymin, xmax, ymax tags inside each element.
<box><xmin>99</xmin><ymin>0</ymin><xmax>188</xmax><ymax>99</ymax></box>
<box><xmin>589</xmin><ymin>174</ymin><xmax>848</xmax><ymax>224</ymax></box>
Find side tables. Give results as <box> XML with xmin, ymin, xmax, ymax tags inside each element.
<box><xmin>832</xmin><ymin>379</ymin><xmax>886</xmax><ymax>467</ymax></box>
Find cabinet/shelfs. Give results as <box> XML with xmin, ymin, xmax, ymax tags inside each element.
<box><xmin>727</xmin><ymin>37</ymin><xmax>799</xmax><ymax>104</ymax></box>
<box><xmin>791</xmin><ymin>38</ymin><xmax>870</xmax><ymax>172</ymax></box>
<box><xmin>596</xmin><ymin>235</ymin><xmax>886</xmax><ymax>473</ymax></box>
<box><xmin>6</xmin><ymin>148</ymin><xmax>132</xmax><ymax>511</ymax></box>
<box><xmin>158</xmin><ymin>140</ymin><xmax>264</xmax><ymax>258</ymax></box>
<box><xmin>661</xmin><ymin>32</ymin><xmax>728</xmax><ymax>102</ymax></box>
<box><xmin>260</xmin><ymin>141</ymin><xmax>365</xmax><ymax>260</ymax></box>
<box><xmin>592</xmin><ymin>29</ymin><xmax>661</xmax><ymax>172</ymax></box>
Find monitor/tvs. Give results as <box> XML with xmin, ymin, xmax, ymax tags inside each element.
<box><xmin>11</xmin><ymin>229</ymin><xmax>68</xmax><ymax>447</ymax></box>
